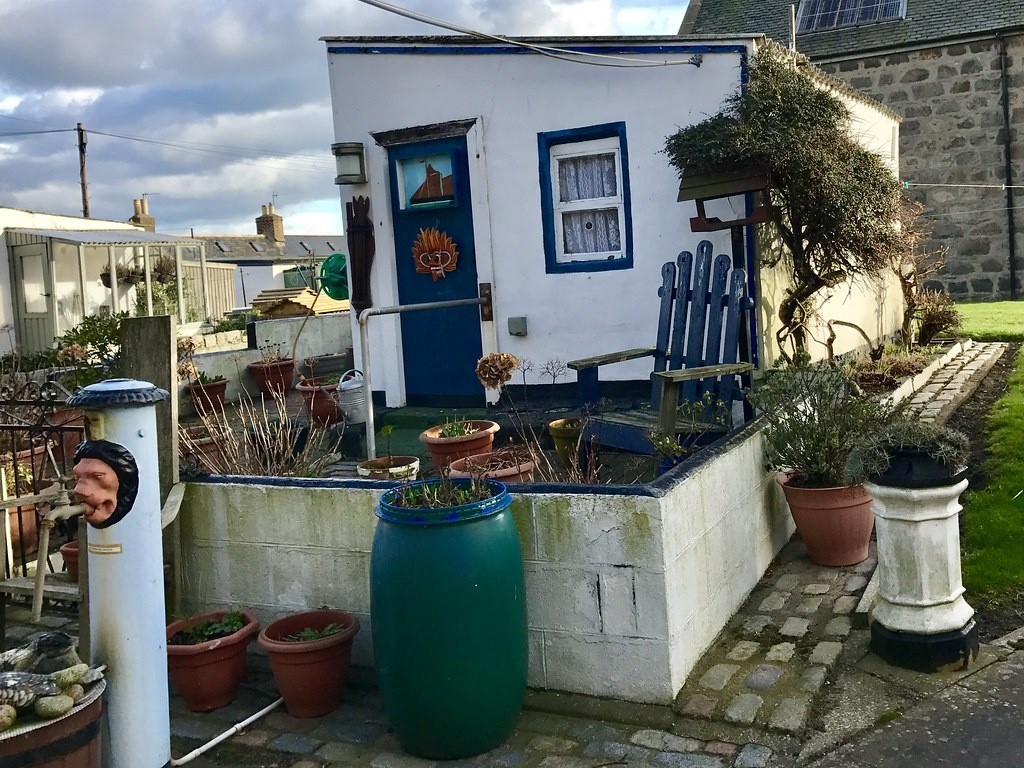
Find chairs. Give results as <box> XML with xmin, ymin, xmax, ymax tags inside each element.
<box><xmin>568</xmin><ymin>241</ymin><xmax>756</xmax><ymax>481</ymax></box>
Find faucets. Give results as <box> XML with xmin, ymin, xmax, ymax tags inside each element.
<box><xmin>29</xmin><ymin>474</ymin><xmax>95</xmax><ymax>625</ymax></box>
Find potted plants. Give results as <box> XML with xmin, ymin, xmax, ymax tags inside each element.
<box><xmin>861</xmin><ymin>419</ymin><xmax>969</xmax><ymax>491</ymax></box>
<box><xmin>166</xmin><ymin>602</ymin><xmax>257</xmax><ymax>710</ymax></box>
<box><xmin>188</xmin><ymin>370</ymin><xmax>228</xmax><ymax>414</ymax></box>
<box><xmin>371</xmin><ymin>464</ymin><xmax>529</xmax><ymax>760</ymax></box>
<box><xmin>446</xmin><ymin>443</ymin><xmax>545</xmax><ymax>483</ymax></box>
<box><xmin>357</xmin><ymin>425</ymin><xmax>421</xmax><ymax>485</ymax></box>
<box><xmin>248</xmin><ymin>339</ymin><xmax>294</xmax><ymax>395</ymax></box>
<box><xmin>34</xmin><ymin>317</ymin><xmax>127</xmax><ymax>462</ymax></box>
<box><xmin>245</xmin><ymin>413</ymin><xmax>311</xmax><ymax>464</ymax></box>
<box><xmin>100</xmin><ymin>256</ymin><xmax>178</xmax><ymax>288</ymax></box>
<box><xmin>178</xmin><ymin>424</ymin><xmax>234</xmax><ymax>476</ymax></box>
<box><xmin>760</xmin><ymin>350</ymin><xmax>890</xmax><ymax>565</ymax></box>
<box><xmin>550</xmin><ymin>414</ymin><xmax>588</xmax><ymax>467</ymax></box>
<box><xmin>0</xmin><ymin>380</ymin><xmax>70</xmax><ymax>493</ymax></box>
<box><xmin>419</xmin><ymin>411</ymin><xmax>498</xmax><ymax>470</ymax></box>
<box><xmin>294</xmin><ymin>373</ymin><xmax>353</xmax><ymax>424</ymax></box>
<box><xmin>0</xmin><ymin>352</ymin><xmax>70</xmax><ymax>482</ymax></box>
<box><xmin>258</xmin><ymin>606</ymin><xmax>360</xmax><ymax>720</ymax></box>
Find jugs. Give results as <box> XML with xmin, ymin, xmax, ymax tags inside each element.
<box><xmin>320</xmin><ymin>369</ymin><xmax>366</xmax><ymax>424</ymax></box>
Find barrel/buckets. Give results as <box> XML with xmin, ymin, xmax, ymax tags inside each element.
<box><xmin>61</xmin><ymin>541</ymin><xmax>78</xmax><ymax>582</ymax></box>
<box><xmin>0</xmin><ymin>695</ymin><xmax>101</xmax><ymax>768</ymax></box>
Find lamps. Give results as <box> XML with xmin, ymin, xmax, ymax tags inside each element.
<box><xmin>333</xmin><ymin>143</ymin><xmax>367</xmax><ymax>185</ymax></box>
